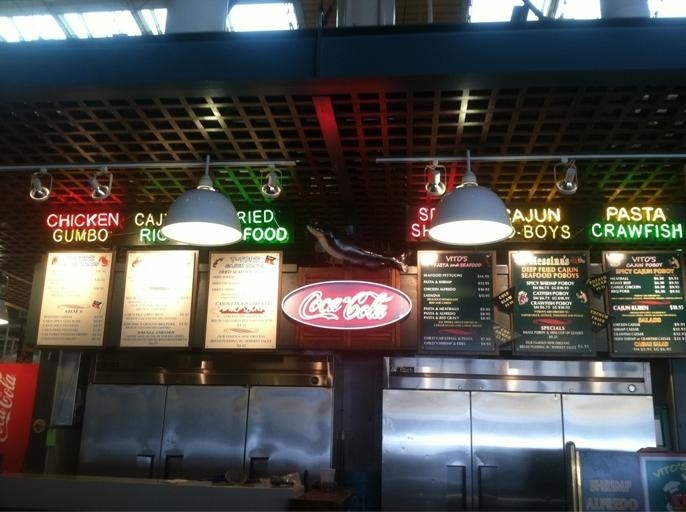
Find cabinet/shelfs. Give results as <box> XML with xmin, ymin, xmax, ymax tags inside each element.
<box><xmin>244</xmin><ymin>387</ymin><xmax>335</xmax><ymax>484</ymax></box>
<box><xmin>382</xmin><ymin>391</ymin><xmax>473</xmax><ymax>512</ymax></box>
<box><xmin>162</xmin><ymin>385</ymin><xmax>247</xmax><ymax>488</ymax></box>
<box><xmin>80</xmin><ymin>384</ymin><xmax>166</xmax><ymax>486</ymax></box>
<box><xmin>467</xmin><ymin>392</ymin><xmax>567</xmax><ymax>512</ymax></box>
<box><xmin>562</xmin><ymin>393</ymin><xmax>656</xmax><ymax>457</ymax></box>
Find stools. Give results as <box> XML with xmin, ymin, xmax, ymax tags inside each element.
<box><xmin>292</xmin><ymin>488</ymin><xmax>352</xmax><ymax>512</ymax></box>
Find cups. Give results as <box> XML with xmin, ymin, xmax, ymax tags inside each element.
<box><xmin>319</xmin><ymin>470</ymin><xmax>336</xmax><ymax>493</ymax></box>
<box><xmin>260</xmin><ymin>478</ymin><xmax>270</xmax><ymax>488</ymax></box>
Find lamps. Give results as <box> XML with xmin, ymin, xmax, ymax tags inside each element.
<box><xmin>424</xmin><ymin>158</ymin><xmax>449</xmax><ymax>198</ymax></box>
<box><xmin>429</xmin><ymin>173</ymin><xmax>515</xmax><ymax>247</ymax></box>
<box><xmin>88</xmin><ymin>166</ymin><xmax>114</xmax><ymax>201</ymax></box>
<box><xmin>29</xmin><ymin>166</ymin><xmax>54</xmax><ymax>205</ymax></box>
<box><xmin>162</xmin><ymin>176</ymin><xmax>242</xmax><ymax>247</ymax></box>
<box><xmin>261</xmin><ymin>166</ymin><xmax>283</xmax><ymax>197</ymax></box>
<box><xmin>550</xmin><ymin>159</ymin><xmax>583</xmax><ymax>195</ymax></box>
